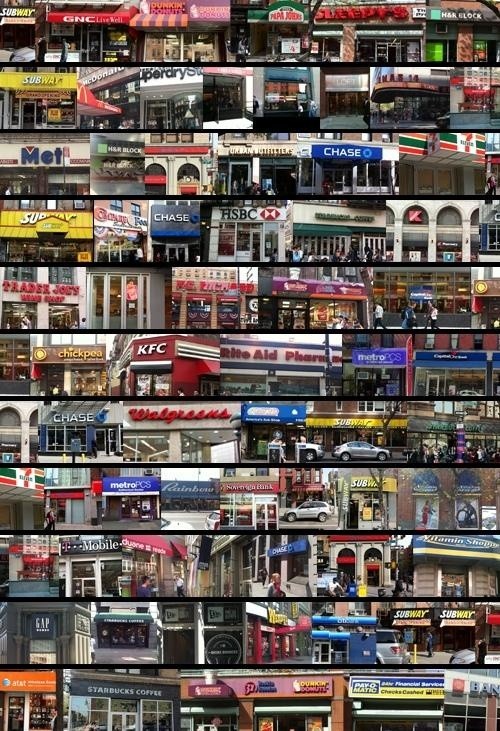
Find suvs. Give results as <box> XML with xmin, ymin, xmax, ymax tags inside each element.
<box><xmin>374</xmin><ymin>629</ymin><xmax>409</xmax><ymax>665</ymax></box>
<box><xmin>285</xmin><ymin>501</ymin><xmax>332</xmax><ymax>521</ymax></box>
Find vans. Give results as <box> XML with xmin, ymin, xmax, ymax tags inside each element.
<box><xmin>204</xmin><ymin>511</ymin><xmax>219</xmax><ymax>530</ymax></box>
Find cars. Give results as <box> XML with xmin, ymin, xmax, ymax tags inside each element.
<box><xmin>450</xmin><ymin>648</ymin><xmax>477</xmax><ymax>665</ymax></box>
<box><xmin>332</xmin><ymin>441</ymin><xmax>392</xmax><ymax>461</ymax></box>
<box><xmin>298</xmin><ymin>443</ymin><xmax>325</xmax><ymax>461</ymax></box>
<box><xmin>8</xmin><ymin>45</ymin><xmax>36</xmax><ymax>62</ymax></box>
